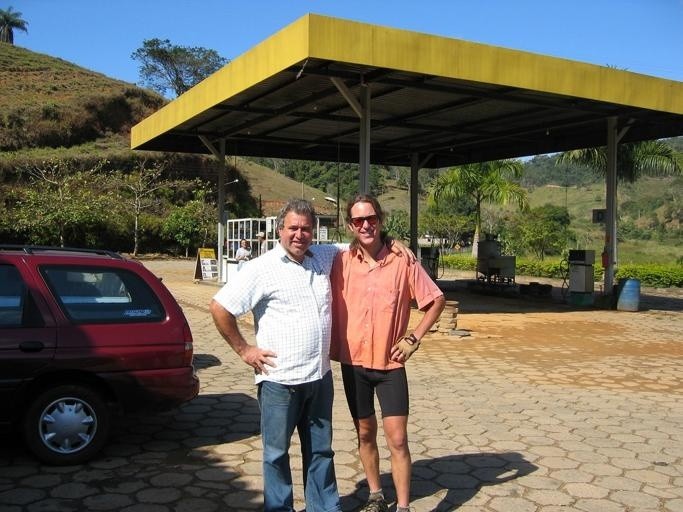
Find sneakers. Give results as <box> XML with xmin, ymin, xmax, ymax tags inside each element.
<box><xmin>360</xmin><ymin>495</ymin><xmax>388</xmax><ymax>512</ymax></box>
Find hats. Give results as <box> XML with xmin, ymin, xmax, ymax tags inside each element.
<box><xmin>255</xmin><ymin>232</ymin><xmax>264</xmax><ymax>238</ymax></box>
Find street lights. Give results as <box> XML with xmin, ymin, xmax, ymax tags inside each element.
<box><xmin>223</xmin><ymin>179</ymin><xmax>240</xmax><ymax>186</ymax></box>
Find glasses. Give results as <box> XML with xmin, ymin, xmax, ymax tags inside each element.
<box><xmin>351</xmin><ymin>216</ymin><xmax>381</xmax><ymax>226</ymax></box>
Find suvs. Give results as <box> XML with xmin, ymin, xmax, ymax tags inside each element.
<box><xmin>0</xmin><ymin>243</ymin><xmax>199</xmax><ymax>466</ymax></box>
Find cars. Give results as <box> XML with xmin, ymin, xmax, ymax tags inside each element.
<box><xmin>418</xmin><ymin>234</ymin><xmax>448</xmax><ymax>249</ymax></box>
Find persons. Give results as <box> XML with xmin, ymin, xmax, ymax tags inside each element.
<box><xmin>234</xmin><ymin>238</ymin><xmax>253</xmax><ymax>270</ymax></box>
<box><xmin>210</xmin><ymin>198</ymin><xmax>415</xmax><ymax>511</ymax></box>
<box><xmin>255</xmin><ymin>231</ymin><xmax>266</xmax><ymax>255</ymax></box>
<box><xmin>328</xmin><ymin>195</ymin><xmax>446</xmax><ymax>512</ymax></box>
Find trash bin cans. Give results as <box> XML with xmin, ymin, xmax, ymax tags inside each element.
<box><xmin>615</xmin><ymin>279</ymin><xmax>640</xmax><ymax>312</ymax></box>
